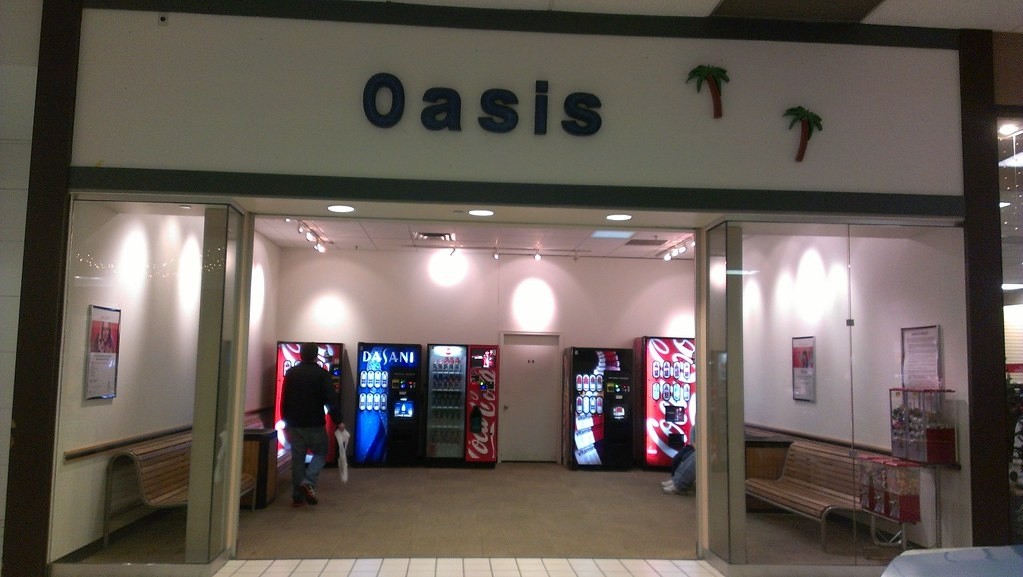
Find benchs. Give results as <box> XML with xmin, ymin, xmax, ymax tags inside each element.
<box><xmin>746</xmin><ymin>440</ymin><xmax>909</xmax><ymax>551</ymax></box>
<box><xmin>103</xmin><ymin>435</ymin><xmax>256</xmax><ymax>552</ymax></box>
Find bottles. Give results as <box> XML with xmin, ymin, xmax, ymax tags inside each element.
<box><xmin>392</xmin><ymin>373</ymin><xmax>416</xmax><ymax>391</ymax></box>
<box><xmin>652</xmin><ymin>360</ymin><xmax>690</xmax><ymax>402</ymax></box>
<box><xmin>432</xmin><ymin>357</ymin><xmax>463</xmax><ymax>443</ymax></box>
<box><xmin>359</xmin><ymin>371</ymin><xmax>388</xmax><ymax>410</ymax></box>
<box><xmin>576</xmin><ymin>375</ymin><xmax>602</xmax><ymax>414</ymax></box>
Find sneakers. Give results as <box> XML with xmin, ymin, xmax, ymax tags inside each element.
<box><xmin>293</xmin><ymin>498</ymin><xmax>305</xmax><ymax>506</ymax></box>
<box><xmin>662</xmin><ymin>479</ymin><xmax>675</xmax><ymax>487</ymax></box>
<box><xmin>300</xmin><ymin>482</ymin><xmax>320</xmax><ymax>505</ymax></box>
<box><xmin>662</xmin><ymin>484</ymin><xmax>689</xmax><ymax>495</ymax></box>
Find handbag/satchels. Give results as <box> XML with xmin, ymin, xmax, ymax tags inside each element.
<box><xmin>334</xmin><ymin>429</ymin><xmax>350</xmax><ymax>483</ymax></box>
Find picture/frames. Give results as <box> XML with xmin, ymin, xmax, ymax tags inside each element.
<box><xmin>793</xmin><ymin>335</ymin><xmax>817</xmax><ymax>401</ymax></box>
<box><xmin>84</xmin><ymin>304</ymin><xmax>122</xmax><ymax>401</ymax></box>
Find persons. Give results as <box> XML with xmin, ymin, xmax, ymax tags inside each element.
<box><xmin>279</xmin><ymin>343</ymin><xmax>345</xmax><ymax>507</ymax></box>
<box><xmin>662</xmin><ymin>414</ymin><xmax>696</xmax><ymax>494</ymax></box>
